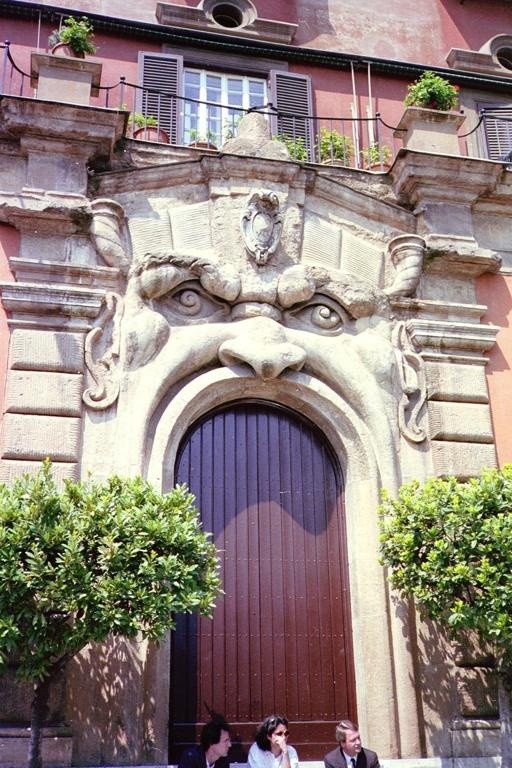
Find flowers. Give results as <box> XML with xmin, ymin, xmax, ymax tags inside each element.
<box><xmin>401</xmin><ymin>71</ymin><xmax>459</xmax><ymax>109</ymax></box>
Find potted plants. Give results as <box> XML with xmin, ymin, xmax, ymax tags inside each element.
<box><xmin>48</xmin><ymin>15</ymin><xmax>94</xmax><ymax>59</ymax></box>
<box><xmin>122</xmin><ymin>103</ymin><xmax>394</xmax><ymax>172</ymax></box>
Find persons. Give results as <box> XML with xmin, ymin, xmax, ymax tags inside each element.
<box><xmin>246</xmin><ymin>714</ymin><xmax>299</xmax><ymax>768</ymax></box>
<box><xmin>174</xmin><ymin>715</ymin><xmax>232</xmax><ymax>768</ymax></box>
<box><xmin>323</xmin><ymin>719</ymin><xmax>381</xmax><ymax>768</ymax></box>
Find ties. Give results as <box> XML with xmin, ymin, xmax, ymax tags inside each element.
<box><xmin>351</xmin><ymin>758</ymin><xmax>356</xmax><ymax>768</ymax></box>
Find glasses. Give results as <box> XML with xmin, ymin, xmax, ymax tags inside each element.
<box><xmin>272</xmin><ymin>730</ymin><xmax>290</xmax><ymax>737</ymax></box>
